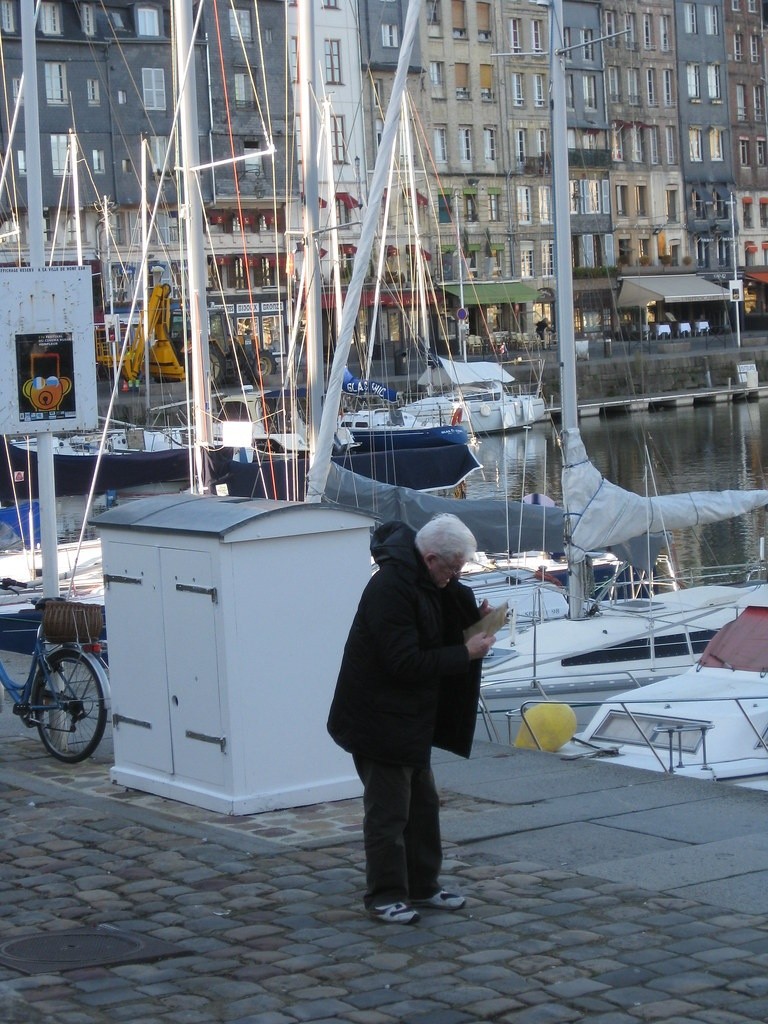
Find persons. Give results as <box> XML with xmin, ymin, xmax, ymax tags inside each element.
<box><xmin>536</xmin><ymin>318</ymin><xmax>552</xmax><ymax>350</ymax></box>
<box><xmin>327</xmin><ymin>513</ymin><xmax>496</xmax><ymax>925</ymax></box>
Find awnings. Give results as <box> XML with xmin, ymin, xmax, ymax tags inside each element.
<box><xmin>745</xmin><ymin>241</ymin><xmax>768</xmax><ymax>253</ymax></box>
<box><xmin>319</xmin><ymin>244</ymin><xmax>432</xmax><ymax>261</ymax></box>
<box><xmin>117</xmin><ymin>313</ymin><xmax>142</xmax><ymax>324</ymax></box>
<box><xmin>0</xmin><ymin>260</ymin><xmax>166</xmax><ymax>276</ymax></box>
<box><xmin>742</xmin><ymin>197</ymin><xmax>768</xmax><ymax>203</ymax></box>
<box><xmin>438</xmin><ymin>283</ymin><xmax>545</xmax><ymax>305</ymax></box>
<box><xmin>617</xmin><ymin>274</ymin><xmax>730</xmax><ymax>308</ymax></box>
<box><xmin>744</xmin><ymin>272</ymin><xmax>768</xmax><ymax>283</ymax></box>
<box><xmin>301</xmin><ymin>188</ymin><xmax>428</xmax><ymax>209</ymax></box>
<box><xmin>611</xmin><ymin>119</ymin><xmax>651</xmax><ymax>129</ymax></box>
<box><xmin>207</xmin><ymin>210</ymin><xmax>280</xmax><ymax>225</ymax></box>
<box><xmin>692</xmin><ymin>183</ymin><xmax>737</xmax><ymax>205</ymax></box>
<box><xmin>697</xmin><ymin>232</ymin><xmax>733</xmax><ymax>242</ymax></box>
<box><xmin>211</xmin><ymin>254</ymin><xmax>285</xmax><ymax>267</ymax></box>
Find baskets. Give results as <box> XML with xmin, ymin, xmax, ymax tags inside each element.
<box><xmin>43</xmin><ymin>601</ymin><xmax>103</xmax><ymax>642</ymax></box>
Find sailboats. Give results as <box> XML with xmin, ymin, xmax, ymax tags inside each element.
<box><xmin>0</xmin><ymin>1</ymin><xmax>768</xmax><ymax>784</ymax></box>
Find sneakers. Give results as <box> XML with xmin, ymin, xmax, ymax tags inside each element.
<box><xmin>407</xmin><ymin>891</ymin><xmax>466</xmax><ymax>909</ymax></box>
<box><xmin>369</xmin><ymin>902</ymin><xmax>421</xmax><ymax>923</ymax></box>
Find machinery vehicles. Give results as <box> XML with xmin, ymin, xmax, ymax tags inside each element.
<box><xmin>120</xmin><ymin>283</ymin><xmax>278</xmax><ymax>389</ymax></box>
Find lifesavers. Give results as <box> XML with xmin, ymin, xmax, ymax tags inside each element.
<box><xmin>535</xmin><ymin>571</ymin><xmax>563</xmax><ymax>586</ymax></box>
<box><xmin>452</xmin><ymin>408</ymin><xmax>463</xmax><ymax>426</ymax></box>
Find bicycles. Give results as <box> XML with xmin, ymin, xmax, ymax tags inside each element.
<box><xmin>1</xmin><ymin>577</ymin><xmax>115</xmax><ymax>763</ymax></box>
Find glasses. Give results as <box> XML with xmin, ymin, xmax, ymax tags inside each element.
<box><xmin>433</xmin><ymin>552</ymin><xmax>462</xmax><ymax>576</ymax></box>
